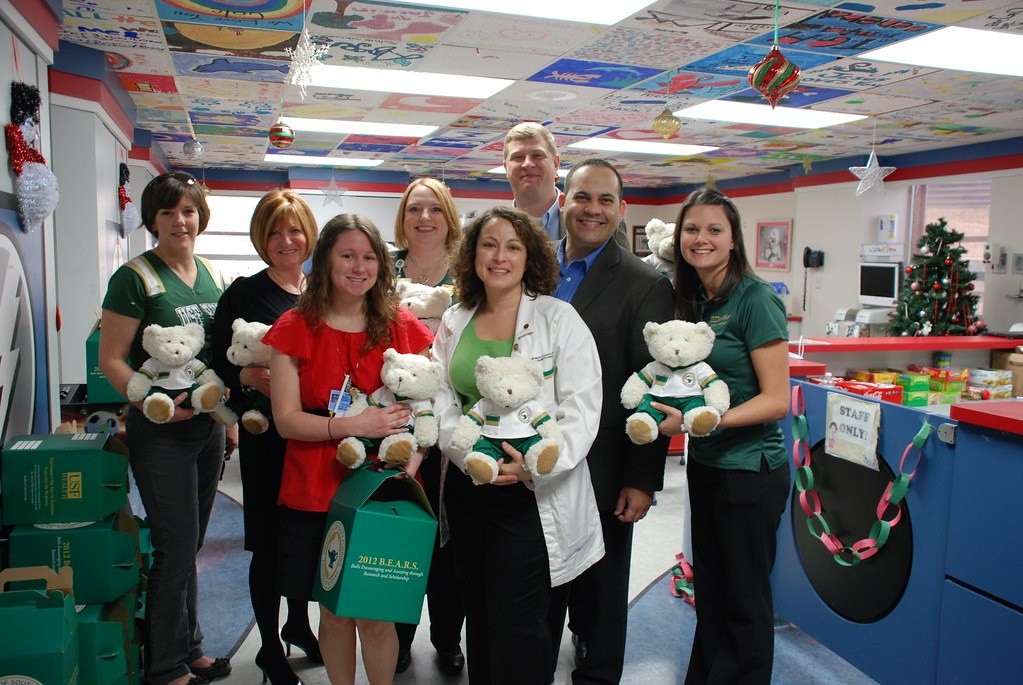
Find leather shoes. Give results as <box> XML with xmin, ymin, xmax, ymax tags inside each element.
<box><xmin>438</xmin><ymin>653</ymin><xmax>465</xmax><ymax>674</ymax></box>
<box><xmin>395</xmin><ymin>650</ymin><xmax>412</xmax><ymax>673</ymax></box>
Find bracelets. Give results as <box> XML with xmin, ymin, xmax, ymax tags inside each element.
<box><xmin>328</xmin><ymin>416</ymin><xmax>335</xmax><ymax>442</ymax></box>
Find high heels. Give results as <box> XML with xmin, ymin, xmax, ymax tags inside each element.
<box><xmin>281</xmin><ymin>622</ymin><xmax>324</xmax><ymax>666</ymax></box>
<box><xmin>256</xmin><ymin>647</ymin><xmax>305</xmax><ymax>685</ymax></box>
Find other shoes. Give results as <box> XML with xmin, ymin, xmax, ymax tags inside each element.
<box><xmin>191</xmin><ymin>657</ymin><xmax>232</xmax><ymax>676</ymax></box>
<box><xmin>186</xmin><ymin>676</ymin><xmax>209</xmax><ymax>685</ymax></box>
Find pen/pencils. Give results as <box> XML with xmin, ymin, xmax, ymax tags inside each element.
<box><xmin>333</xmin><ymin>373</ymin><xmax>351</xmax><ymax>419</ymax></box>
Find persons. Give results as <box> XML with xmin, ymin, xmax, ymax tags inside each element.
<box><xmin>209</xmin><ymin>190</ymin><xmax>324</xmax><ymax>685</ymax></box>
<box><xmin>670</xmin><ymin>187</ymin><xmax>791</xmax><ymax>685</ymax></box>
<box><xmin>385</xmin><ymin>122</ymin><xmax>672</xmax><ymax>685</ymax></box>
<box><xmin>432</xmin><ymin>207</ymin><xmax>606</xmax><ymax>684</ymax></box>
<box><xmin>260</xmin><ymin>214</ymin><xmax>435</xmax><ymax>685</ymax></box>
<box><xmin>99</xmin><ymin>173</ymin><xmax>237</xmax><ymax>685</ymax></box>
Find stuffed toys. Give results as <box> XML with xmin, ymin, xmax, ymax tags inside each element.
<box><xmin>621</xmin><ymin>320</ymin><xmax>731</xmax><ymax>445</ymax></box>
<box><xmin>445</xmin><ymin>355</ymin><xmax>566</xmax><ymax>484</ymax></box>
<box><xmin>226</xmin><ymin>318</ymin><xmax>273</xmax><ymax>435</ymax></box>
<box><xmin>639</xmin><ymin>218</ymin><xmax>681</xmax><ymax>288</ymax></box>
<box><xmin>126</xmin><ymin>323</ymin><xmax>238</xmax><ymax>429</ymax></box>
<box><xmin>335</xmin><ymin>348</ymin><xmax>438</xmax><ymax>470</ymax></box>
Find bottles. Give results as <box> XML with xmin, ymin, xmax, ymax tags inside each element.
<box><xmin>934</xmin><ymin>352</ymin><xmax>952</xmax><ymax>370</ymax></box>
<box><xmin>984</xmin><ymin>245</ymin><xmax>990</xmax><ymax>260</ymax></box>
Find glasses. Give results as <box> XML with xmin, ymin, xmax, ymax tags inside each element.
<box><xmin>149</xmin><ymin>173</ymin><xmax>195</xmax><ymax>188</ymax></box>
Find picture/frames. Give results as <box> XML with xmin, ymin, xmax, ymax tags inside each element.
<box><xmin>1011</xmin><ymin>253</ymin><xmax>1023</xmax><ymax>273</ymax></box>
<box><xmin>633</xmin><ymin>224</ymin><xmax>651</xmax><ymax>257</ymax></box>
<box><xmin>757</xmin><ymin>220</ymin><xmax>792</xmax><ymax>271</ymax></box>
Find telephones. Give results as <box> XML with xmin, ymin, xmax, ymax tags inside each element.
<box><xmin>804</xmin><ymin>247</ymin><xmax>824</xmax><ymax>268</ymax></box>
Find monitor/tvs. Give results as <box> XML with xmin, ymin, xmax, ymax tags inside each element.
<box><xmin>858</xmin><ymin>262</ymin><xmax>899</xmax><ymax>307</ymax></box>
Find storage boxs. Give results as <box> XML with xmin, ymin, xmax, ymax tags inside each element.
<box><xmin>313</xmin><ymin>460</ymin><xmax>439</xmax><ymax>626</ymax></box>
<box><xmin>809</xmin><ymin>350</ymin><xmax>1023</xmax><ymax>406</ymax></box>
<box><xmin>0</xmin><ymin>513</ymin><xmax>155</xmax><ymax>685</ymax></box>
<box><xmin>85</xmin><ymin>318</ymin><xmax>130</xmax><ymax>406</ymax></box>
<box><xmin>1</xmin><ymin>425</ymin><xmax>131</xmax><ymax>525</ymax></box>
